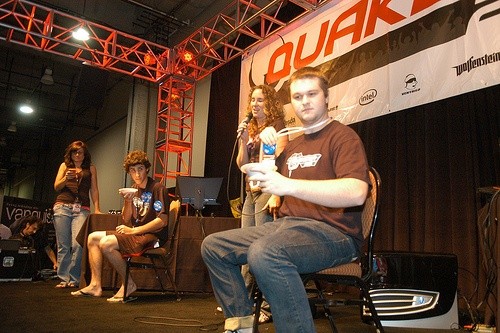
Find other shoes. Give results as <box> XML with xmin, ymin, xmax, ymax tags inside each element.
<box><xmin>224</xmin><ymin>328</ymin><xmax>253</xmax><ymax>333</ymax></box>
<box><xmin>56</xmin><ymin>280</ymin><xmax>78</xmax><ymax>287</ymax></box>
<box><xmin>259</xmin><ymin>302</ymin><xmax>272</xmax><ymax>323</ymax></box>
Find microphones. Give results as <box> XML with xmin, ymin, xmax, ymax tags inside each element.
<box><xmin>237</xmin><ymin>112</ymin><xmax>253</xmax><ymax>138</ymax></box>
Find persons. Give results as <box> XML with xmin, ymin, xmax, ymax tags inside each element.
<box><xmin>70</xmin><ymin>151</ymin><xmax>169</xmax><ymax>304</ymax></box>
<box><xmin>11</xmin><ymin>140</ymin><xmax>101</xmax><ymax>289</ymax></box>
<box><xmin>200</xmin><ymin>67</ymin><xmax>373</xmax><ymax>333</ymax></box>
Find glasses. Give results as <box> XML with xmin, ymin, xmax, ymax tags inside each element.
<box><xmin>71</xmin><ymin>150</ymin><xmax>83</xmax><ymax>155</ymax></box>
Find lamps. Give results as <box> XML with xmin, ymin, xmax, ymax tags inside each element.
<box><xmin>18</xmin><ymin>99</ymin><xmax>34</xmax><ymax>115</ymax></box>
<box><xmin>7</xmin><ymin>120</ymin><xmax>16</xmax><ymax>132</ymax></box>
<box><xmin>40</xmin><ymin>63</ymin><xmax>54</xmax><ymax>85</ymax></box>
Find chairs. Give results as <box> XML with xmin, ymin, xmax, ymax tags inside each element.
<box><xmin>0</xmin><ymin>236</ymin><xmax>45</xmax><ymax>283</ymax></box>
<box><xmin>112</xmin><ymin>193</ymin><xmax>182</xmax><ymax>305</ymax></box>
<box><xmin>252</xmin><ymin>167</ymin><xmax>384</xmax><ymax>333</ymax></box>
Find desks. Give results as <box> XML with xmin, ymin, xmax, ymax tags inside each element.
<box><xmin>76</xmin><ymin>213</ymin><xmax>240</xmax><ymax>293</ymax></box>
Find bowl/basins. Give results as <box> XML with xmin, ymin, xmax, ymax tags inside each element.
<box><xmin>240</xmin><ymin>162</ymin><xmax>278</xmax><ymax>177</ymax></box>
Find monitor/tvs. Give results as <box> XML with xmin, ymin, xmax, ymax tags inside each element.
<box><xmin>175</xmin><ymin>175</ymin><xmax>223</xmax><ymax>210</ymax></box>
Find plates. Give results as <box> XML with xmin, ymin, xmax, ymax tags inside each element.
<box><xmin>119</xmin><ymin>188</ymin><xmax>138</xmax><ymax>194</ymax></box>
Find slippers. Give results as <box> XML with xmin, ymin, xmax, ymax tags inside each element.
<box><xmin>109</xmin><ymin>294</ymin><xmax>136</xmax><ymax>303</ymax></box>
<box><xmin>74</xmin><ymin>289</ymin><xmax>98</xmax><ymax>298</ymax></box>
